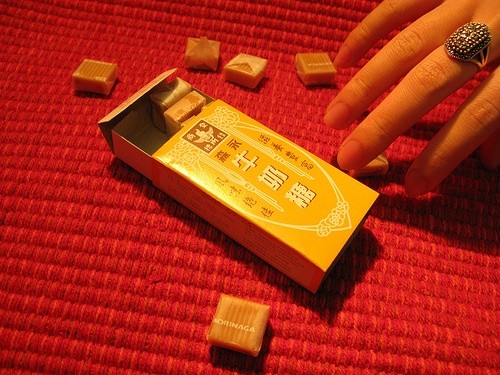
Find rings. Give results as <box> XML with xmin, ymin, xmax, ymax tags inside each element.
<box><xmin>444</xmin><ymin>21</ymin><xmax>493</xmax><ymax>72</ymax></box>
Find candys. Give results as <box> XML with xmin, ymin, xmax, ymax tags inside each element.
<box><xmin>71</xmin><ymin>38</ymin><xmax>389</xmax><ymax>357</ymax></box>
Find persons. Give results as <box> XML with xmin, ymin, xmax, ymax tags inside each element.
<box><xmin>324</xmin><ymin>0</ymin><xmax>500</xmax><ymax>195</ymax></box>
